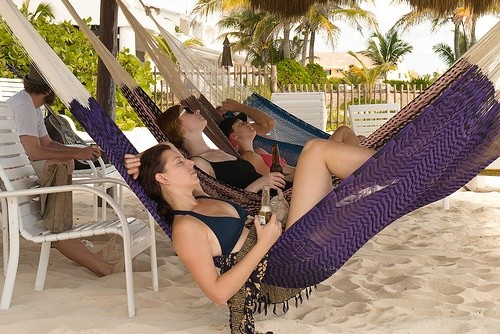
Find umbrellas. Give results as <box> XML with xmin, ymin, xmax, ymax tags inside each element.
<box><xmin>221</xmin><ymin>35</ymin><xmax>234</xmax><ymax>67</ymax></box>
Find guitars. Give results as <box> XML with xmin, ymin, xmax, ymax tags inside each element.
<box><xmin>6</xmin><ymin>62</ymin><xmax>97</xmax><ymax>170</ymax></box>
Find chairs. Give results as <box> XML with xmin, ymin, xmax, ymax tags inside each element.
<box><xmin>0</xmin><ymin>102</ymin><xmax>160</xmax><ymax>319</ymax></box>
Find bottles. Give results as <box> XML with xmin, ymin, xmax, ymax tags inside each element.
<box><xmin>269</xmin><ymin>142</ymin><xmax>283</xmax><ymax>196</ymax></box>
<box><xmin>257</xmin><ymin>184</ymin><xmax>273</xmax><ymax>227</ymax></box>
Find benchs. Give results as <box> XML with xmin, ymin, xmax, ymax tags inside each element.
<box><xmin>271</xmin><ymin>92</ymin><xmax>328</xmax><ymax>134</ymax></box>
<box><xmin>348</xmin><ymin>104</ymin><xmax>403</xmax><ymax>140</ymax></box>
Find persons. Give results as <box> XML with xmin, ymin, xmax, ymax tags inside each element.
<box><xmin>124</xmin><ymin>139</ymin><xmax>378</xmax><ymax>306</ymax></box>
<box><xmin>0</xmin><ymin>61</ymin><xmax>135</xmax><ymax>277</ymax></box>
<box><xmin>155</xmin><ymin>98</ymin><xmax>367</xmax><ymax>196</ymax></box>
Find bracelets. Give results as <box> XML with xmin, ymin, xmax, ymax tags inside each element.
<box><xmin>242</xmin><ymin>105</ymin><xmax>250</xmax><ymax>114</ymax></box>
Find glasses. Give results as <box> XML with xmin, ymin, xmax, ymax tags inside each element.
<box><xmin>178</xmin><ymin>106</ymin><xmax>193</xmax><ymax>120</ymax></box>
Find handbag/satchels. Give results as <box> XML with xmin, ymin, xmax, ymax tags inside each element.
<box><xmin>38</xmin><ymin>163</ymin><xmax>73</xmax><ymax>233</ymax></box>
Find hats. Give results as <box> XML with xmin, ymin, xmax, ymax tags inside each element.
<box><xmin>221</xmin><ymin>111</ymin><xmax>247</xmax><ymax>132</ymax></box>
<box><xmin>25</xmin><ymin>63</ymin><xmax>48</xmax><ymax>86</ymax></box>
